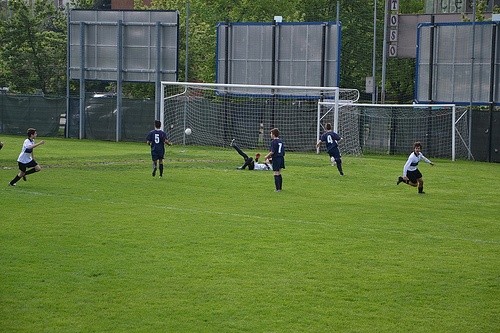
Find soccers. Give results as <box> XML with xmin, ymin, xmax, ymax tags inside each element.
<box><xmin>185</xmin><ymin>128</ymin><xmax>192</xmax><ymax>135</ymax></box>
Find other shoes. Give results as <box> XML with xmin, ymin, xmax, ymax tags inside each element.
<box><xmin>152</xmin><ymin>173</ymin><xmax>155</xmax><ymax>177</ymax></box>
<box><xmin>340</xmin><ymin>173</ymin><xmax>344</xmax><ymax>176</ymax></box>
<box><xmin>22</xmin><ymin>173</ymin><xmax>26</xmax><ymax>181</ymax></box>
<box><xmin>230</xmin><ymin>139</ymin><xmax>235</xmax><ymax>147</ymax></box>
<box><xmin>237</xmin><ymin>166</ymin><xmax>243</xmax><ymax>170</ymax></box>
<box><xmin>331</xmin><ymin>157</ymin><xmax>335</xmax><ymax>167</ymax></box>
<box><xmin>397</xmin><ymin>176</ymin><xmax>402</xmax><ymax>185</ymax></box>
<box><xmin>418</xmin><ymin>191</ymin><xmax>425</xmax><ymax>194</ymax></box>
<box><xmin>8</xmin><ymin>182</ymin><xmax>16</xmax><ymax>187</ymax></box>
<box><xmin>159</xmin><ymin>176</ymin><xmax>162</xmax><ymax>179</ymax></box>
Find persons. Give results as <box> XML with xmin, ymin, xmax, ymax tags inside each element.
<box><xmin>396</xmin><ymin>143</ymin><xmax>433</xmax><ymax>194</ymax></box>
<box><xmin>316</xmin><ymin>124</ymin><xmax>344</xmax><ymax>176</ymax></box>
<box><xmin>8</xmin><ymin>128</ymin><xmax>45</xmax><ymax>186</ymax></box>
<box><xmin>147</xmin><ymin>120</ymin><xmax>172</xmax><ymax>178</ymax></box>
<box><xmin>265</xmin><ymin>128</ymin><xmax>286</xmax><ymax>191</ymax></box>
<box><xmin>230</xmin><ymin>139</ymin><xmax>273</xmax><ymax>171</ymax></box>
